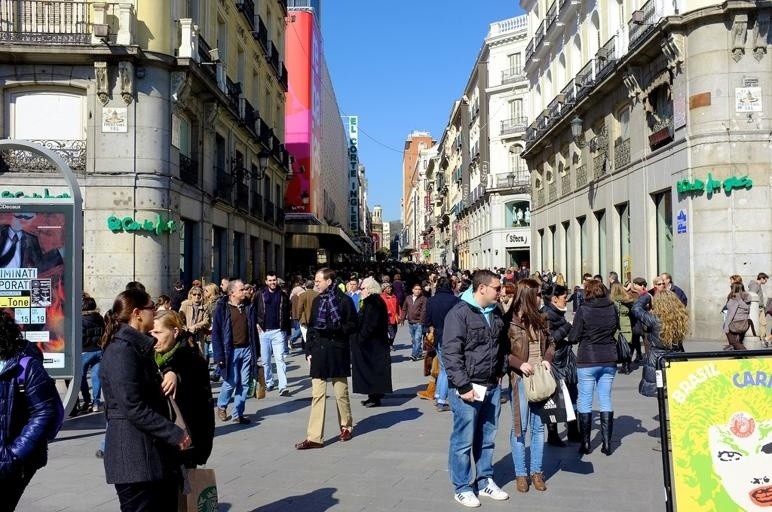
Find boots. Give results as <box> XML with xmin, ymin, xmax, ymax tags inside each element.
<box><xmin>417</xmin><ymin>380</ymin><xmax>438</xmax><ymax>401</ymax></box>
<box><xmin>566</xmin><ymin>419</ymin><xmax>581</xmax><ymax>444</ymax></box>
<box><xmin>578</xmin><ymin>412</ymin><xmax>593</xmax><ymax>455</ymax></box>
<box><xmin>599</xmin><ymin>411</ymin><xmax>614</xmax><ymax>456</ymax></box>
<box><xmin>546</xmin><ymin>422</ymin><xmax>567</xmax><ymax>449</ymax></box>
<box><xmin>618</xmin><ymin>361</ymin><xmax>632</xmax><ymax>375</ymax></box>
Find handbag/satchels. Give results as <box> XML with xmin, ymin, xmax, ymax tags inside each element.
<box><xmin>522</xmin><ymin>359</ymin><xmax>557</xmax><ymax>404</ymax></box>
<box><xmin>614</xmin><ymin>332</ymin><xmax>634</xmax><ymax>364</ymax></box>
<box><xmin>248</xmin><ymin>364</ymin><xmax>268</xmax><ymax>399</ymax></box>
<box><xmin>181</xmin><ymin>468</ymin><xmax>221</xmax><ymax>512</ymax></box>
<box><xmin>728</xmin><ymin>319</ymin><xmax>749</xmax><ymax>334</ymax></box>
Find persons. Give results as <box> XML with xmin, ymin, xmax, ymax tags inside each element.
<box><xmin>0</xmin><ymin>212</ymin><xmax>64</xmax><ymax>331</ymax></box>
<box><xmin>513</xmin><ymin>206</ymin><xmax>531</xmax><ymax>226</ymax></box>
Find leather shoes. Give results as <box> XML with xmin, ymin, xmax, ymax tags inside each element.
<box><xmin>532</xmin><ymin>473</ymin><xmax>547</xmax><ymax>492</ymax></box>
<box><xmin>338</xmin><ymin>427</ymin><xmax>353</xmax><ymax>443</ymax></box>
<box><xmin>516</xmin><ymin>476</ymin><xmax>531</xmax><ymax>493</ymax></box>
<box><xmin>294</xmin><ymin>438</ymin><xmax>325</xmax><ymax>450</ymax></box>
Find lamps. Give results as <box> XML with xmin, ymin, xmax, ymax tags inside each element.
<box><xmin>570</xmin><ymin>114</ymin><xmax>609</xmax><ymax>159</ymax></box>
<box><xmin>229</xmin><ymin>145</ymin><xmax>271</xmax><ymax>192</ymax></box>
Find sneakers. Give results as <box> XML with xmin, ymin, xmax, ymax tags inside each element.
<box><xmin>763</xmin><ymin>334</ymin><xmax>772</xmax><ymax>349</ymax></box>
<box><xmin>279</xmin><ymin>390</ymin><xmax>293</xmax><ymax>397</ymax></box>
<box><xmin>436</xmin><ymin>403</ymin><xmax>451</xmax><ymax>414</ymax></box>
<box><xmin>288</xmin><ymin>338</ymin><xmax>297</xmax><ymax>352</ymax></box>
<box><xmin>232</xmin><ymin>417</ymin><xmax>253</xmax><ymax>426</ymax></box>
<box><xmin>266</xmin><ymin>385</ymin><xmax>276</xmax><ymax>392</ymax></box>
<box><xmin>453</xmin><ymin>490</ymin><xmax>481</xmax><ymax>510</ymax></box>
<box><xmin>215</xmin><ymin>408</ymin><xmax>230</xmax><ymax>422</ymax></box>
<box><xmin>80</xmin><ymin>402</ymin><xmax>100</xmax><ymax>414</ymax></box>
<box><xmin>409</xmin><ymin>353</ymin><xmax>424</xmax><ymax>362</ymax></box>
<box><xmin>478</xmin><ymin>478</ymin><xmax>509</xmax><ymax>501</ymax></box>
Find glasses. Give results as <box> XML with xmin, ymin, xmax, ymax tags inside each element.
<box><xmin>192</xmin><ymin>293</ymin><xmax>201</xmax><ymax>297</ymax></box>
<box><xmin>313</xmin><ymin>280</ymin><xmax>322</xmax><ymax>284</ymax></box>
<box><xmin>477</xmin><ymin>282</ymin><xmax>501</xmax><ymax>292</ymax></box>
<box><xmin>655</xmin><ymin>282</ymin><xmax>666</xmax><ymax>287</ymax></box>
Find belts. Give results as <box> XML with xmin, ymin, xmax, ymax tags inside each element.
<box><xmin>231</xmin><ymin>343</ymin><xmax>250</xmax><ymax>349</ymax></box>
